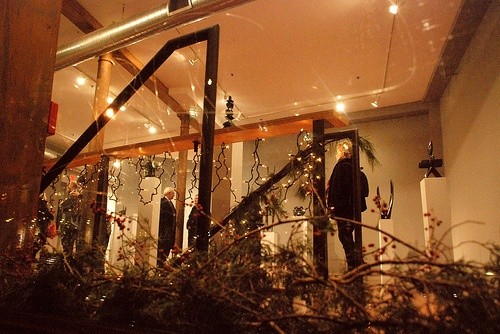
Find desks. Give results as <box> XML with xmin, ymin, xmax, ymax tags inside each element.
<box><xmin>419</xmin><ymin>176</ymin><xmax>453</xmax><ymax>274</ymax></box>
<box><xmin>378</xmin><ymin>218</ymin><xmax>397</xmax><ymax>286</ymax></box>
<box><xmin>258</xmin><ymin>231</ymin><xmax>281</xmax><ymax>279</ymax></box>
<box><xmin>301</xmin><ymin>217</ymin><xmax>316</xmax><ymax>274</ymax></box>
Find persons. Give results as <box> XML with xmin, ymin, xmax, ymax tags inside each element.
<box><xmin>187</xmin><ymin>194</ymin><xmax>199</xmax><ymax>249</ymax></box>
<box><xmin>328</xmin><ymin>149</ymin><xmax>369</xmax><ymax>273</ymax></box>
<box><xmin>32</xmin><ymin>192</ymin><xmax>55</xmax><ymax>263</ymax></box>
<box><xmin>157</xmin><ymin>187</ymin><xmax>177</xmax><ymax>268</ymax></box>
<box><xmin>56</xmin><ymin>191</ymin><xmax>80</xmax><ymax>261</ymax></box>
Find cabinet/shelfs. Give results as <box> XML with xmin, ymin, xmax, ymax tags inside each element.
<box><xmin>113</xmin><ymin>212</ymin><xmax>150</xmax><ymax>276</ymax></box>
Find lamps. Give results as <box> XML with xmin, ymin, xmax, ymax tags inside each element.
<box><xmin>221</xmin><ymin>96</ymin><xmax>237</xmax><ymax>127</ymax></box>
